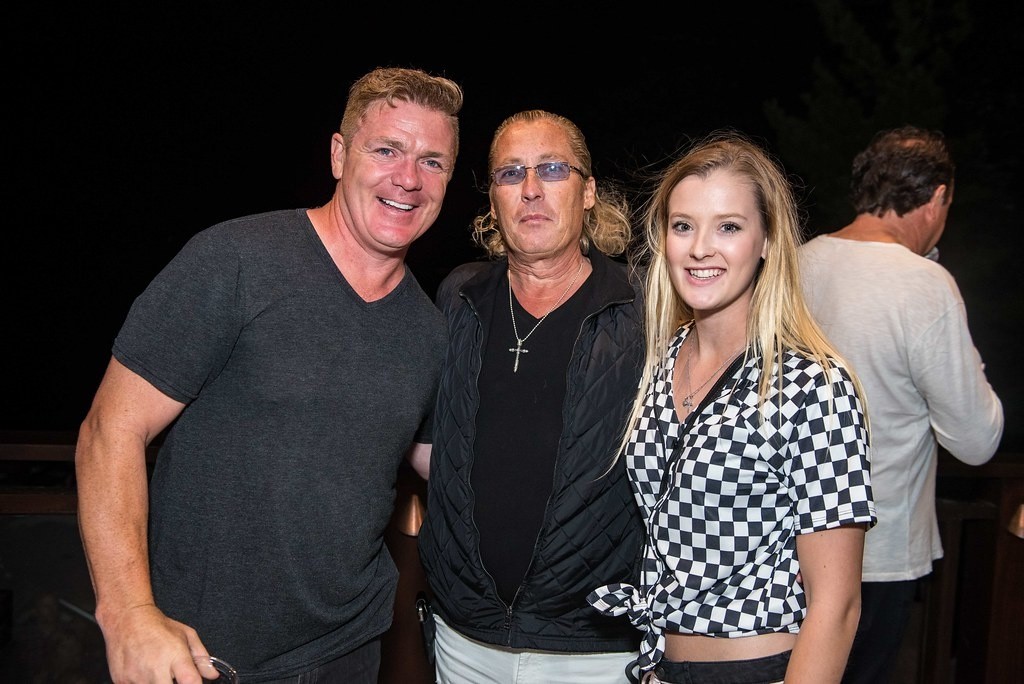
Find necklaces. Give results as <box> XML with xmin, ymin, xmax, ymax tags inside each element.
<box><xmin>506</xmin><ymin>260</ymin><xmax>584</xmax><ymax>372</ymax></box>
<box><xmin>682</xmin><ymin>326</ymin><xmax>747</xmax><ymax>413</ymax></box>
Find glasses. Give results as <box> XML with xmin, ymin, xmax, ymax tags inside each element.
<box><xmin>489</xmin><ymin>162</ymin><xmax>586</xmax><ymax>187</ymax></box>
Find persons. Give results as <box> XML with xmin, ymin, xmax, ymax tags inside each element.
<box><xmin>422</xmin><ymin>112</ymin><xmax>652</xmax><ymax>684</ymax></box>
<box><xmin>792</xmin><ymin>129</ymin><xmax>1006</xmax><ymax>684</ymax></box>
<box><xmin>597</xmin><ymin>148</ymin><xmax>879</xmax><ymax>684</ymax></box>
<box><xmin>72</xmin><ymin>67</ymin><xmax>462</xmax><ymax>684</ymax></box>
